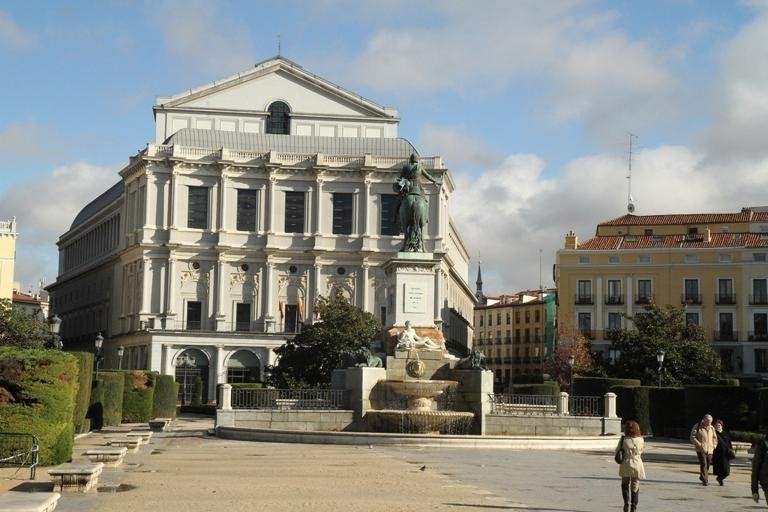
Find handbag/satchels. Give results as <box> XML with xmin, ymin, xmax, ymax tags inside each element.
<box><xmin>615</xmin><ymin>436</ymin><xmax>625</xmax><ymax>464</ymax></box>
<box><xmin>728</xmin><ymin>449</ymin><xmax>736</xmax><ymax>460</ymax></box>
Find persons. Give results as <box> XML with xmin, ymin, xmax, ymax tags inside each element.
<box><xmin>712</xmin><ymin>418</ymin><xmax>733</xmax><ymax>485</ymax></box>
<box><xmin>389</xmin><ymin>151</ymin><xmax>443</xmax><ymax>225</ymax></box>
<box><xmin>614</xmin><ymin>420</ymin><xmax>647</xmax><ymax>512</ymax></box>
<box><xmin>751</xmin><ymin>433</ymin><xmax>768</xmax><ymax>507</ymax></box>
<box><xmin>689</xmin><ymin>414</ymin><xmax>718</xmax><ymax>485</ymax></box>
<box><xmin>398</xmin><ymin>320</ymin><xmax>443</xmax><ymax>350</ymax></box>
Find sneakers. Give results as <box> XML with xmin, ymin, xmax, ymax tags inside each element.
<box><xmin>716</xmin><ymin>477</ymin><xmax>723</xmax><ymax>486</ymax></box>
<box><xmin>699</xmin><ymin>476</ymin><xmax>708</xmax><ymax>486</ymax></box>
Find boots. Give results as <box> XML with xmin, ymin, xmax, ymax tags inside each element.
<box><xmin>622</xmin><ymin>484</ymin><xmax>629</xmax><ymax>512</ymax></box>
<box><xmin>630</xmin><ymin>487</ymin><xmax>639</xmax><ymax>512</ymax></box>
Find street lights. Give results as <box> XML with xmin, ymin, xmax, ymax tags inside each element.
<box><xmin>116</xmin><ymin>345</ymin><xmax>125</xmax><ymax>368</ymax></box>
<box><xmin>568</xmin><ymin>355</ymin><xmax>575</xmax><ymax>396</ymax></box>
<box><xmin>656</xmin><ymin>348</ymin><xmax>666</xmax><ymax>386</ymax></box>
<box><xmin>94</xmin><ymin>332</ymin><xmax>104</xmax><ymax>380</ymax></box>
